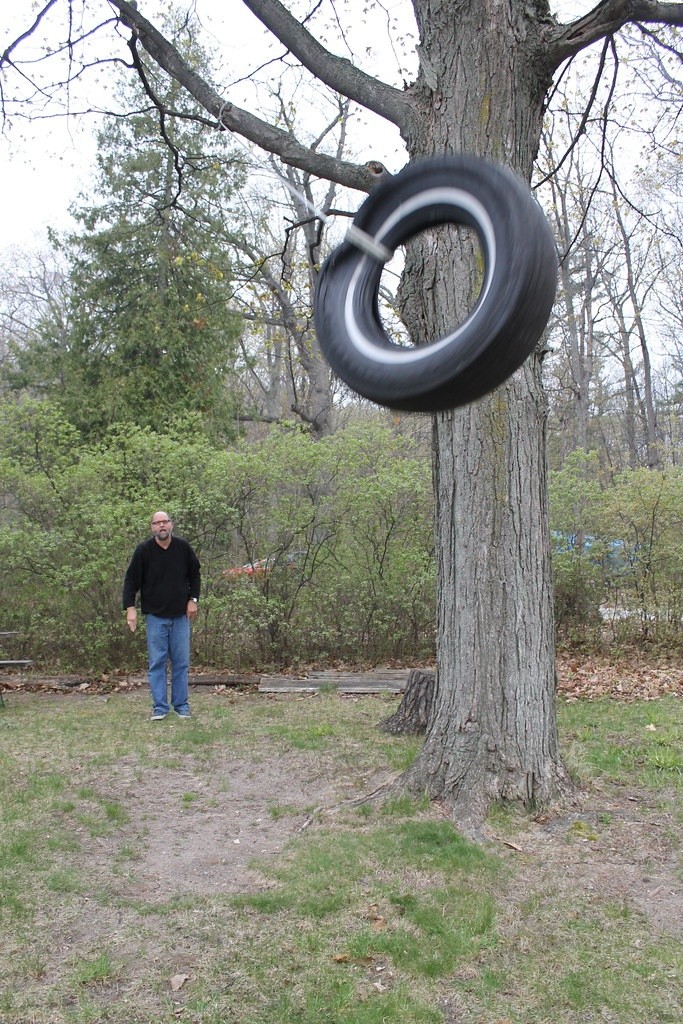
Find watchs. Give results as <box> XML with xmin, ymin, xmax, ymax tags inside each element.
<box><xmin>190</xmin><ymin>598</ymin><xmax>198</xmax><ymax>603</ymax></box>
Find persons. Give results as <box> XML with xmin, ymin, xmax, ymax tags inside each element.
<box><xmin>122</xmin><ymin>511</ymin><xmax>201</xmax><ymax>720</ymax></box>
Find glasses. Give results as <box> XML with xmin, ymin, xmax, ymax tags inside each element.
<box><xmin>152</xmin><ymin>520</ymin><xmax>172</xmax><ymax>525</ymax></box>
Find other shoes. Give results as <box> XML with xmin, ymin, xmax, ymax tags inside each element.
<box><xmin>150</xmin><ymin>709</ymin><xmax>168</xmax><ymax>721</ymax></box>
<box><xmin>176</xmin><ymin>711</ymin><xmax>192</xmax><ymax>719</ymax></box>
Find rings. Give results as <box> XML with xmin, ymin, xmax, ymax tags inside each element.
<box><xmin>128</xmin><ymin>622</ymin><xmax>130</xmax><ymax>624</ymax></box>
